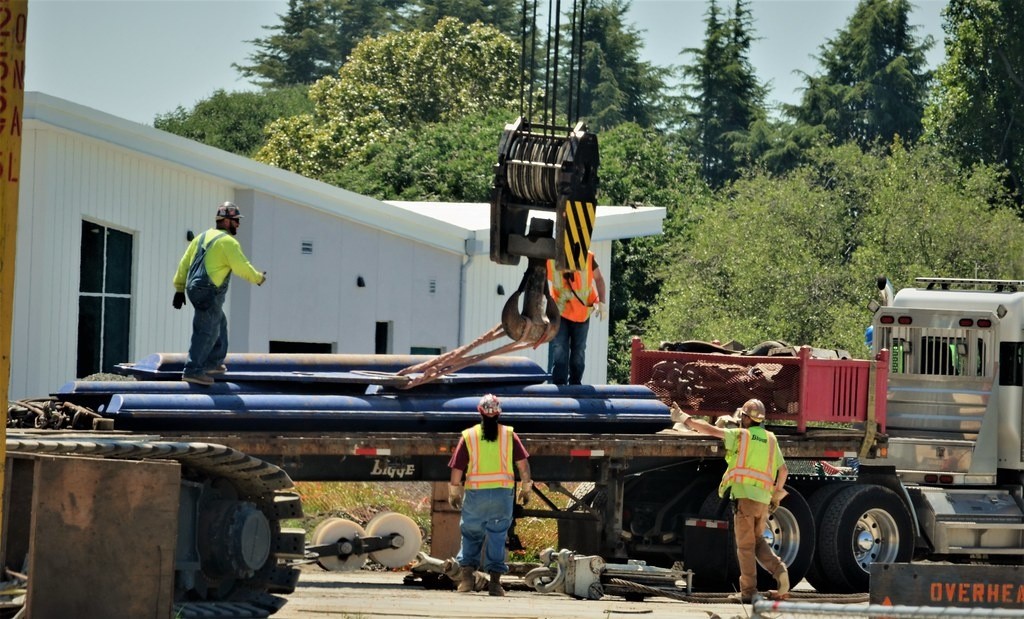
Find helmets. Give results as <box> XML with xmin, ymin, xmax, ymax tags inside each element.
<box><xmin>215</xmin><ymin>201</ymin><xmax>244</xmax><ymax>221</ymax></box>
<box><xmin>736</xmin><ymin>399</ymin><xmax>766</xmax><ymax>422</ymax></box>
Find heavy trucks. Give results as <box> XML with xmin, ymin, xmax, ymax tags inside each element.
<box><xmin>7</xmin><ymin>277</ymin><xmax>1022</xmax><ymax>600</ymax></box>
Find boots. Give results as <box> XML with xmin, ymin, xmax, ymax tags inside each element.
<box><xmin>457</xmin><ymin>566</ymin><xmax>474</xmax><ymax>592</ymax></box>
<box><xmin>488</xmin><ymin>571</ymin><xmax>505</xmax><ymax>596</ymax></box>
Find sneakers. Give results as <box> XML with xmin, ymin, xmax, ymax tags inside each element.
<box><xmin>208</xmin><ymin>364</ymin><xmax>227</xmax><ymax>374</ymax></box>
<box><xmin>181</xmin><ymin>373</ymin><xmax>215</xmax><ymax>385</ymax></box>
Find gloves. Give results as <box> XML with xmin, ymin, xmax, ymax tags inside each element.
<box><xmin>257</xmin><ymin>272</ymin><xmax>266</xmax><ymax>286</ymax></box>
<box><xmin>173</xmin><ymin>292</ymin><xmax>186</xmax><ymax>309</ymax></box>
<box><xmin>594</xmin><ymin>302</ymin><xmax>608</xmax><ymax>322</ymax></box>
<box><xmin>518</xmin><ymin>479</ymin><xmax>533</xmax><ymax>506</ymax></box>
<box><xmin>670</xmin><ymin>401</ymin><xmax>692</xmax><ymax>425</ymax></box>
<box><xmin>448</xmin><ymin>483</ymin><xmax>464</xmax><ymax>511</ymax></box>
<box><xmin>768</xmin><ymin>487</ymin><xmax>788</xmax><ymax>513</ymax></box>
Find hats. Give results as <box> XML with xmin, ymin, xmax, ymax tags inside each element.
<box><xmin>477</xmin><ymin>393</ymin><xmax>502</xmax><ymax>417</ymax></box>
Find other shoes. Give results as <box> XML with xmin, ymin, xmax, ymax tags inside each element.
<box><xmin>727</xmin><ymin>592</ymin><xmax>742</xmax><ymax>599</ymax></box>
<box><xmin>777</xmin><ymin>569</ymin><xmax>790</xmax><ymax>595</ymax></box>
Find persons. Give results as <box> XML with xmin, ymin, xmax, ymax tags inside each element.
<box><xmin>448</xmin><ymin>393</ymin><xmax>532</xmax><ymax>596</ymax></box>
<box><xmin>864</xmin><ymin>325</ymin><xmax>873</xmax><ymax>351</ymax></box>
<box><xmin>546</xmin><ymin>250</ymin><xmax>605</xmax><ymax>386</ymax></box>
<box><xmin>173</xmin><ymin>202</ymin><xmax>269</xmax><ymax>385</ymax></box>
<box><xmin>668</xmin><ymin>399</ymin><xmax>791</xmax><ymax>603</ymax></box>
